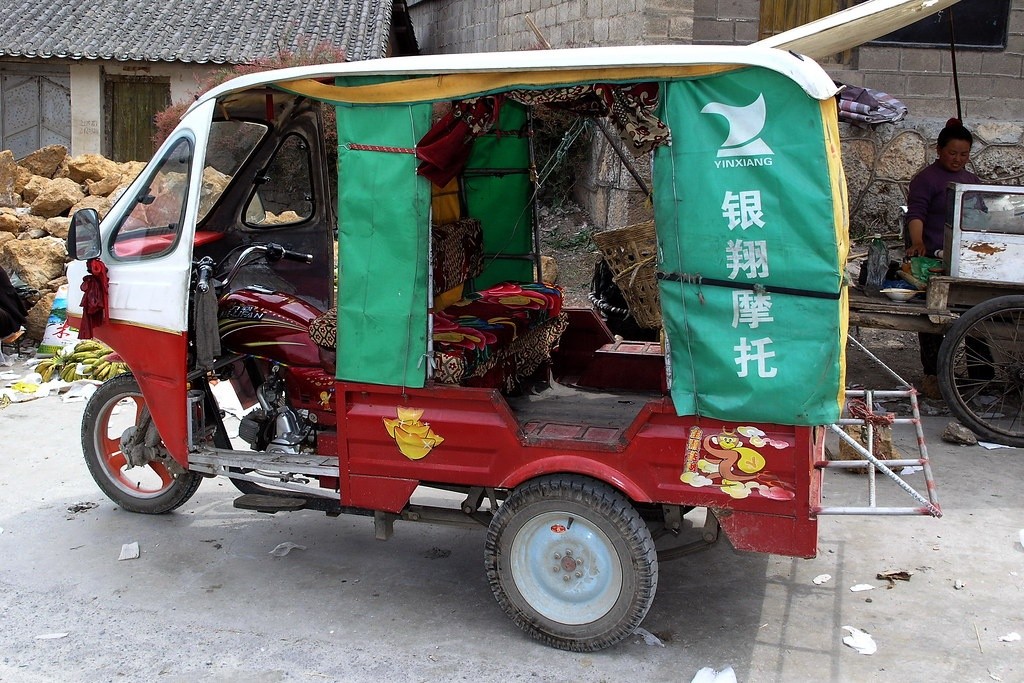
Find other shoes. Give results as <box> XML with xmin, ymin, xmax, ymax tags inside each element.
<box><xmin>970</xmin><ymin>381</ymin><xmax>997</xmax><ymax>397</ymax></box>
<box><xmin>921</xmin><ymin>374</ymin><xmax>942</xmax><ymax>400</ymax></box>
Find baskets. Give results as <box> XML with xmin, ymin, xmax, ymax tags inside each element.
<box><xmin>590</xmin><ymin>219</ymin><xmax>663</xmax><ymax>331</ymax></box>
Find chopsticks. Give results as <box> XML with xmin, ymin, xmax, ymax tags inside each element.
<box><xmin>879</xmin><ymin>290</ymin><xmax>926</xmax><ymax>293</ymax></box>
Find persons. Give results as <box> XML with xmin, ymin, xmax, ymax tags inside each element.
<box><xmin>905</xmin><ymin>117</ymin><xmax>1003</xmax><ymax>402</ymax></box>
<box><xmin>0</xmin><ymin>265</ymin><xmax>29</xmax><ymax>340</ymax></box>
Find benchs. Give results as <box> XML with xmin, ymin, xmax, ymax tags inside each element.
<box><xmin>432</xmin><ymin>218</ymin><xmax>569</xmax><ymax>410</ymax></box>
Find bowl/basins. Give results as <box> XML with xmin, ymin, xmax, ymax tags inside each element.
<box><xmin>883</xmin><ymin>288</ymin><xmax>916</xmax><ymax>303</ymax></box>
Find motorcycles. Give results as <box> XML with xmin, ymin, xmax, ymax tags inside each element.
<box><xmin>64</xmin><ymin>43</ymin><xmax>851</xmax><ymax>654</ymax></box>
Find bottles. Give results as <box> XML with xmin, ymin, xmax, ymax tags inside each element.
<box><xmin>872</xmin><ymin>233</ymin><xmax>882</xmax><ymax>249</ymax></box>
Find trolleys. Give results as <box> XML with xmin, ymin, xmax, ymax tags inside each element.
<box><xmin>849</xmin><ymin>274</ymin><xmax>1024</xmax><ymax>448</ymax></box>
